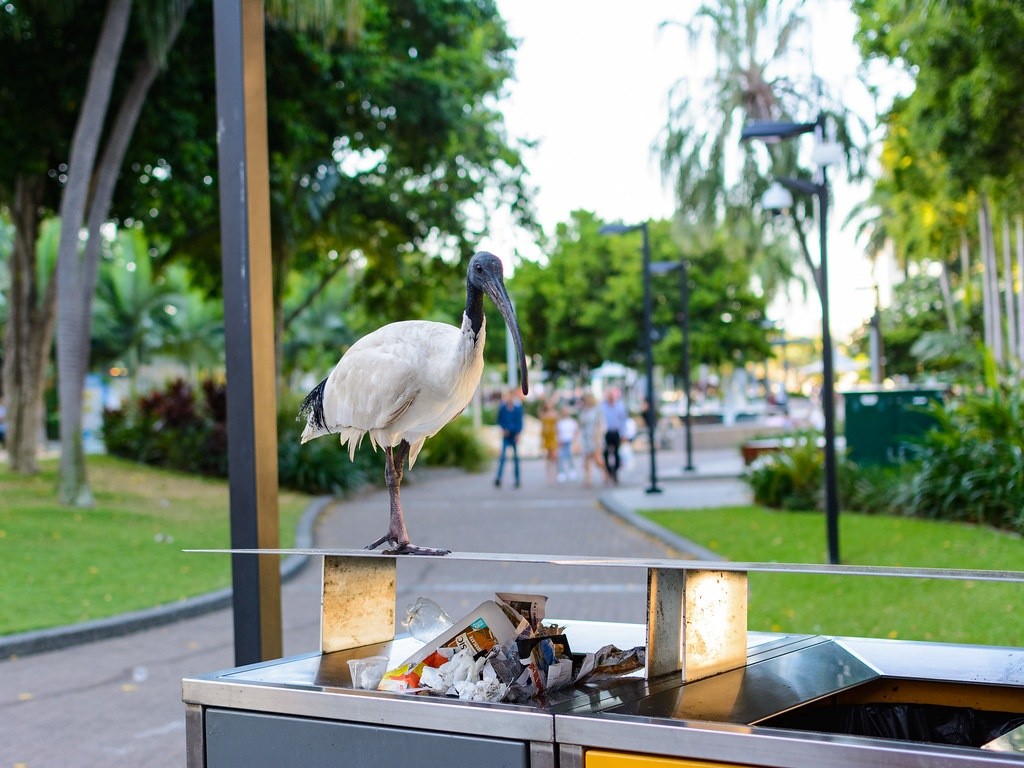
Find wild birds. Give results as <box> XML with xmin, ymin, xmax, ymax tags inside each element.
<box><xmin>295</xmin><ymin>251</ymin><xmax>528</xmax><ymax>556</ymax></box>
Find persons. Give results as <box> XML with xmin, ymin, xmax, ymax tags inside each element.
<box><xmin>537</xmin><ymin>386</ymin><xmax>633</xmax><ymax>490</ymax></box>
<box><xmin>495</xmin><ymin>391</ymin><xmax>522</xmax><ymax>488</ymax></box>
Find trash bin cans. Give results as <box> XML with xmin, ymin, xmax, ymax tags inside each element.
<box><xmin>181</xmin><ymin>554</ymin><xmax>1023</xmax><ymax>768</ymax></box>
